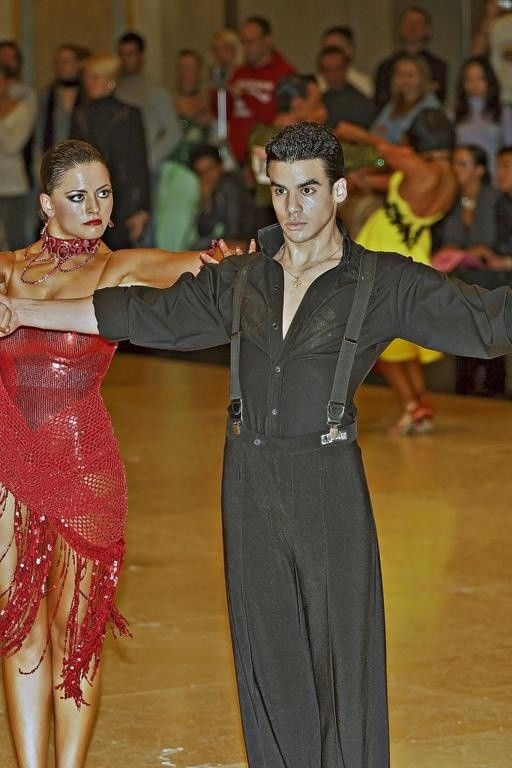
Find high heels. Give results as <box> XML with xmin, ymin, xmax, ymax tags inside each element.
<box><xmin>389</xmin><ymin>401</ymin><xmax>436</xmax><ymax>435</ymax></box>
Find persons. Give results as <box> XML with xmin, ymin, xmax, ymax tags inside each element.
<box><xmin>0</xmin><ymin>1</ymin><xmax>509</xmax><ymax>396</ymax></box>
<box><xmin>0</xmin><ymin>141</ymin><xmax>260</xmax><ymax>767</ymax></box>
<box><xmin>0</xmin><ymin>122</ymin><xmax>512</xmax><ymax>767</ymax></box>
<box><xmin>336</xmin><ymin>105</ymin><xmax>461</xmax><ymax>433</ymax></box>
